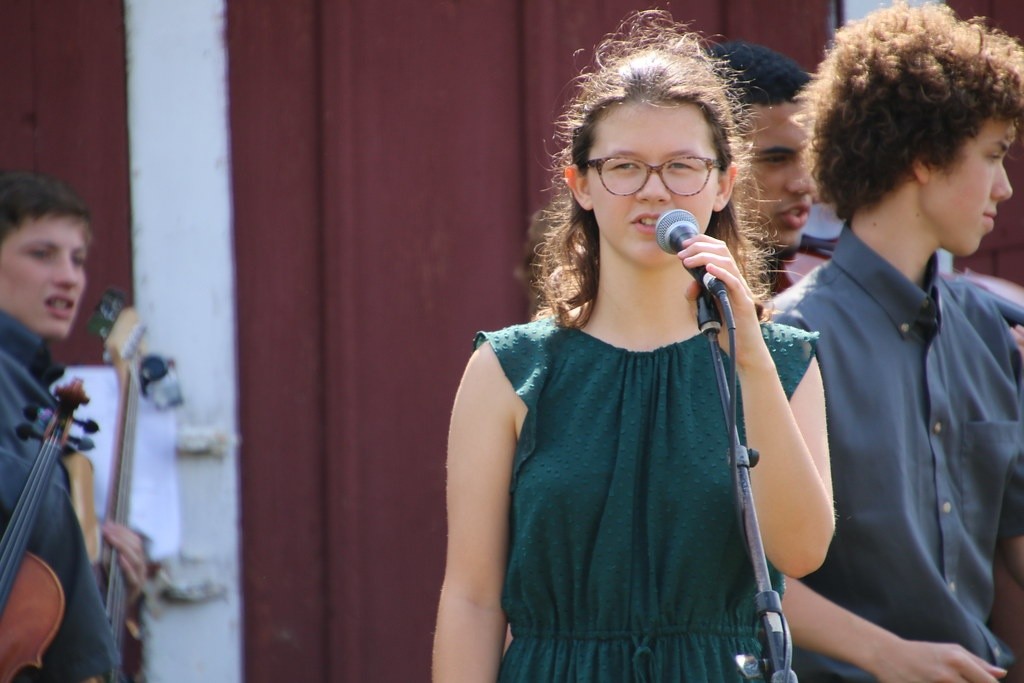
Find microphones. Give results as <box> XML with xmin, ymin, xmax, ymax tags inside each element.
<box><xmin>657</xmin><ymin>210</ymin><xmax>727</xmax><ymax>301</ymax></box>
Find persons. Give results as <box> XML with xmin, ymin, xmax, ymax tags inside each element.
<box><xmin>704</xmin><ymin>41</ymin><xmax>815</xmax><ymax>292</ymax></box>
<box><xmin>0</xmin><ymin>169</ymin><xmax>144</xmax><ymax>683</ymax></box>
<box><xmin>432</xmin><ymin>12</ymin><xmax>837</xmax><ymax>683</ymax></box>
<box><xmin>759</xmin><ymin>0</ymin><xmax>1024</xmax><ymax>683</ymax></box>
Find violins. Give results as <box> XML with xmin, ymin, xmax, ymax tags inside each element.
<box><xmin>91</xmin><ymin>292</ymin><xmax>168</xmax><ymax>682</ymax></box>
<box><xmin>774</xmin><ymin>198</ymin><xmax>1024</xmax><ymax>349</ymax></box>
<box><xmin>0</xmin><ymin>374</ymin><xmax>101</xmax><ymax>683</ymax></box>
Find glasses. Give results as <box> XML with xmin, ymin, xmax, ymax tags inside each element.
<box><xmin>587</xmin><ymin>151</ymin><xmax>720</xmax><ymax>198</ymax></box>
